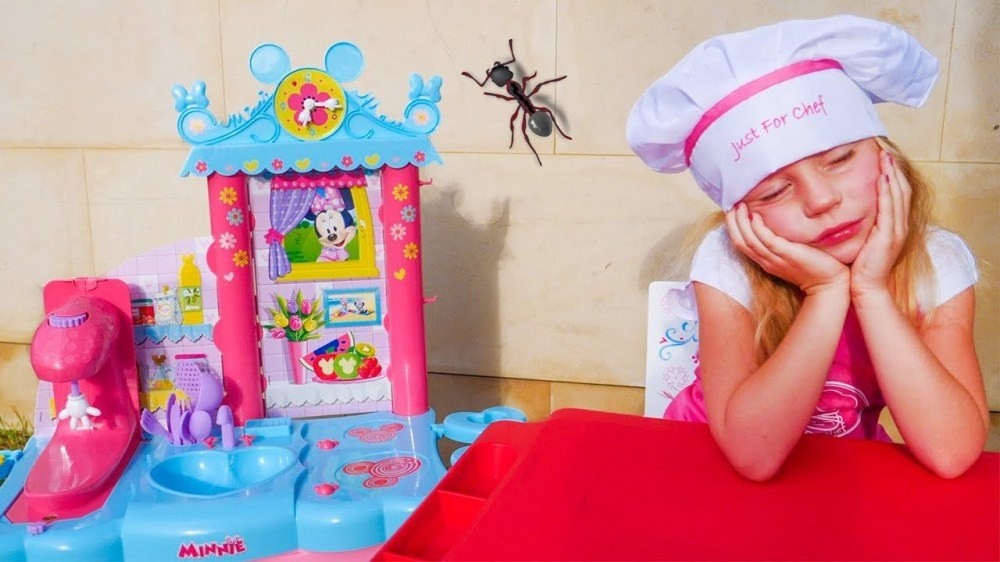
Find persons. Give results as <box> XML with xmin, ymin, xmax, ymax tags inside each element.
<box><xmin>625</xmin><ymin>14</ymin><xmax>989</xmax><ymax>482</ymax></box>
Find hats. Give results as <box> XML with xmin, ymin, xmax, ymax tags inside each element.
<box><xmin>624</xmin><ymin>15</ymin><xmax>939</xmax><ymax>212</ymax></box>
